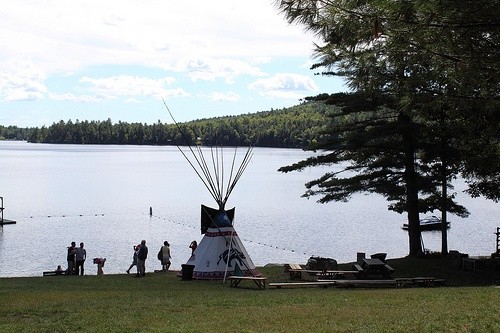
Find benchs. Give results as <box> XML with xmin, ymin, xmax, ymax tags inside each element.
<box><xmin>353</xmin><ymin>264</ymin><xmax>367</xmax><ymax>274</ymax></box>
<box><xmin>289</xmin><ymin>269</ymin><xmax>359</xmax><ymax>281</ymax></box>
<box><xmin>43</xmin><ymin>271</ymin><xmax>67</xmax><ymax>276</ymax></box>
<box><xmin>396</xmin><ymin>277</ymin><xmax>437</xmax><ymax>289</ymax></box>
<box><xmin>384</xmin><ymin>264</ymin><xmax>395</xmax><ymax>275</ymax></box>
<box><xmin>228</xmin><ymin>275</ymin><xmax>267</xmax><ymax>290</ymax></box>
<box><xmin>288</xmin><ymin>264</ymin><xmax>302</xmax><ymax>279</ymax></box>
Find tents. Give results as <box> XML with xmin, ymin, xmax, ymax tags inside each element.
<box><xmin>177</xmin><ymin>208</ymin><xmax>262</xmax><ymax>280</ymax></box>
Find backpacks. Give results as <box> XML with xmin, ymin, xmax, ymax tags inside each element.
<box><xmin>158</xmin><ymin>250</ymin><xmax>163</xmax><ymax>260</ymax></box>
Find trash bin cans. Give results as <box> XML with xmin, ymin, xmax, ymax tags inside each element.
<box><xmin>182</xmin><ymin>264</ymin><xmax>195</xmax><ymax>281</ymax></box>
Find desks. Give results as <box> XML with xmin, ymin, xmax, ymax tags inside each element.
<box><xmin>361</xmin><ymin>259</ymin><xmax>385</xmax><ymax>271</ymax></box>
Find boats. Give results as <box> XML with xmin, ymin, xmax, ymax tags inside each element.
<box><xmin>402</xmin><ymin>215</ymin><xmax>450</xmax><ymax>231</ymax></box>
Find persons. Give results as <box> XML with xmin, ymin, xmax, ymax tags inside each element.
<box><xmin>134</xmin><ymin>240</ymin><xmax>148</xmax><ymax>277</ymax></box>
<box><xmin>70</xmin><ymin>242</ymin><xmax>87</xmax><ymax>275</ymax></box>
<box><xmin>189</xmin><ymin>240</ymin><xmax>197</xmax><ymax>255</ymax></box>
<box><xmin>67</xmin><ymin>241</ymin><xmax>78</xmax><ymax>276</ymax></box>
<box><xmin>126</xmin><ymin>244</ymin><xmax>141</xmax><ymax>275</ymax></box>
<box><xmin>160</xmin><ymin>241</ymin><xmax>171</xmax><ymax>270</ymax></box>
